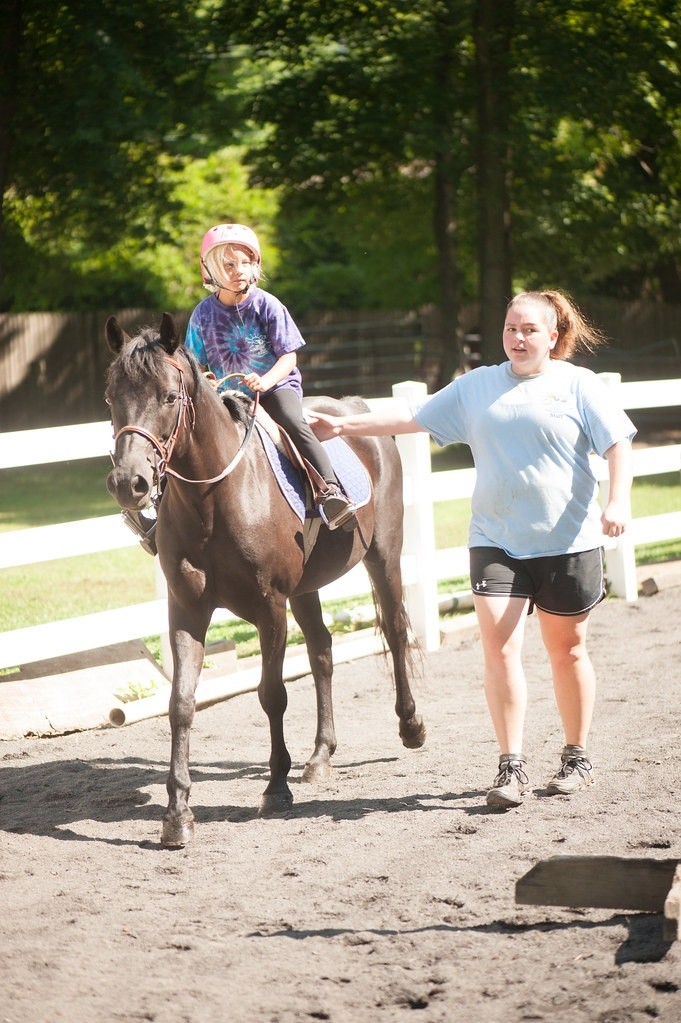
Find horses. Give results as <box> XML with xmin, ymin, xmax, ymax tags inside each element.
<box><xmin>103</xmin><ymin>312</ymin><xmax>427</xmax><ymax>845</ymax></box>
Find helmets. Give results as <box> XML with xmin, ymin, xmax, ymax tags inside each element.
<box><xmin>199</xmin><ymin>223</ymin><xmax>260</xmax><ymax>282</ymax></box>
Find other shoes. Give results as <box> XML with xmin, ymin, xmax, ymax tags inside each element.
<box><xmin>123</xmin><ymin>507</ymin><xmax>158</xmax><ymax>557</ymax></box>
<box><xmin>320</xmin><ymin>484</ymin><xmax>352</xmax><ymax>528</ymax></box>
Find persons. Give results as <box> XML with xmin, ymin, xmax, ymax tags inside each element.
<box><xmin>183</xmin><ymin>223</ymin><xmax>360</xmax><ymax>532</ymax></box>
<box><xmin>306</xmin><ymin>290</ymin><xmax>638</xmax><ymax>805</ymax></box>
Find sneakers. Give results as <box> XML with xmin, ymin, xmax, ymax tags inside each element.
<box><xmin>546</xmin><ymin>745</ymin><xmax>592</xmax><ymax>793</ymax></box>
<box><xmin>485</xmin><ymin>754</ymin><xmax>532</xmax><ymax>804</ymax></box>
